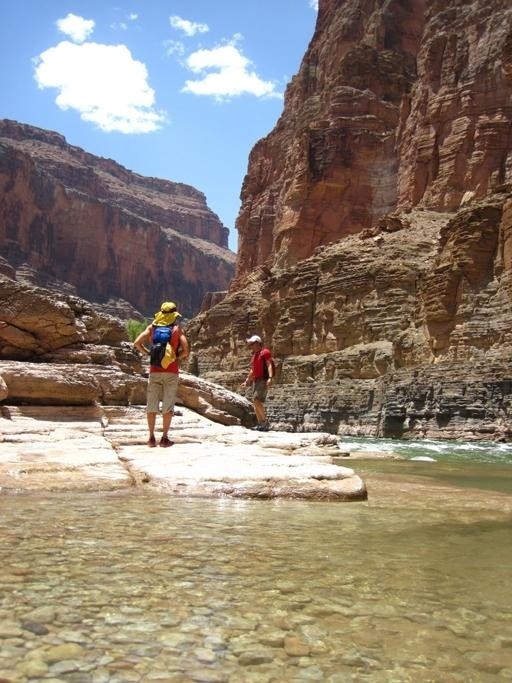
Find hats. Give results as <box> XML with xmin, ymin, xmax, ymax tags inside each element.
<box><xmin>161</xmin><ymin>342</ymin><xmax>176</xmax><ymax>369</ymax></box>
<box><xmin>152</xmin><ymin>302</ymin><xmax>182</xmax><ymax>327</ymax></box>
<box><xmin>246</xmin><ymin>335</ymin><xmax>261</xmax><ymax>343</ymax></box>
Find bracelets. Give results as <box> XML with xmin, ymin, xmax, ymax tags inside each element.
<box><xmin>268</xmin><ymin>377</ymin><xmax>272</xmax><ymax>379</ymax></box>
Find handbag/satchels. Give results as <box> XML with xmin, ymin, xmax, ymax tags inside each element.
<box><xmin>264</xmin><ymin>363</ymin><xmax>275</xmax><ymax>377</ymax></box>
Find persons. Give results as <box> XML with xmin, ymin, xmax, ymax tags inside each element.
<box><xmin>134</xmin><ymin>301</ymin><xmax>189</xmax><ymax>447</ymax></box>
<box><xmin>240</xmin><ymin>335</ymin><xmax>273</xmax><ymax>432</ymax></box>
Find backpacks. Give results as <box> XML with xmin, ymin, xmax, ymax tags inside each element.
<box><xmin>149</xmin><ymin>327</ymin><xmax>173</xmax><ymax>369</ymax></box>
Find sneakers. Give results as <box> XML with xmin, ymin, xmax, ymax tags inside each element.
<box><xmin>145</xmin><ymin>439</ymin><xmax>155</xmax><ymax>447</ymax></box>
<box><xmin>160</xmin><ymin>437</ymin><xmax>174</xmax><ymax>447</ymax></box>
<box><xmin>251</xmin><ymin>421</ymin><xmax>269</xmax><ymax>431</ymax></box>
